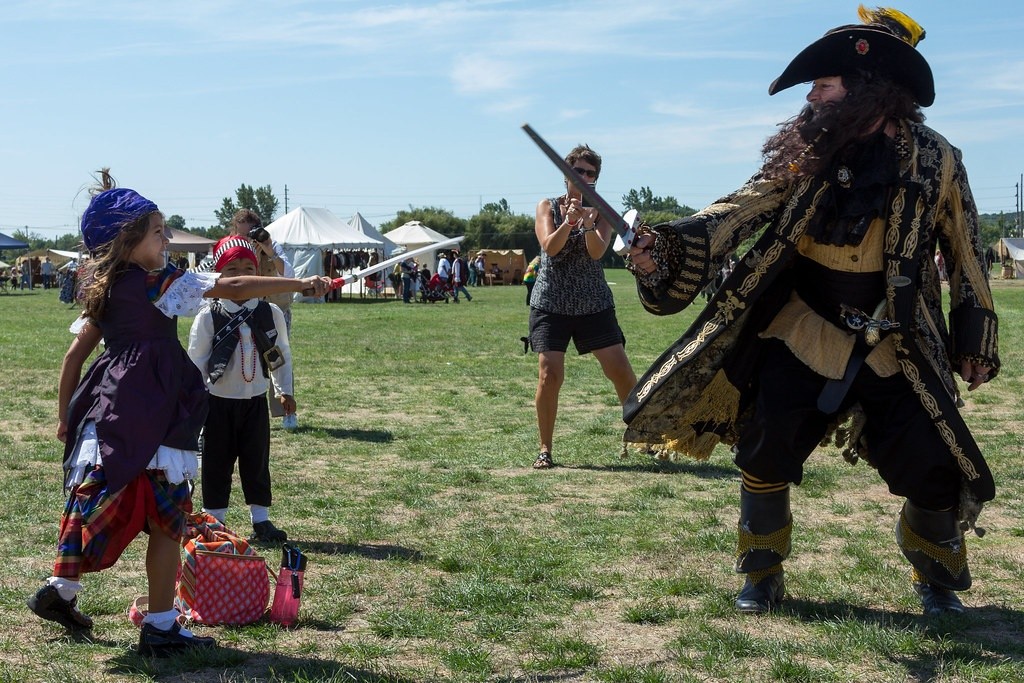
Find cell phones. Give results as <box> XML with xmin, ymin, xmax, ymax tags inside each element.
<box><xmin>581</xmin><ymin>183</ymin><xmax>596</xmax><ymax>209</ymax></box>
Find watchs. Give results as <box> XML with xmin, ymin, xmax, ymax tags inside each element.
<box><xmin>584</xmin><ymin>223</ymin><xmax>595</xmax><ymax>232</ymax></box>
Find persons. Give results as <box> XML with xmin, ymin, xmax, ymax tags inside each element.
<box><xmin>935</xmin><ymin>249</ymin><xmax>943</xmax><ymax>279</ymax></box>
<box><xmin>468</xmin><ymin>254</ymin><xmax>487</xmax><ymax>287</ymax></box>
<box><xmin>228</xmin><ymin>208</ymin><xmax>296</xmax><ymax>429</ymax></box>
<box><xmin>437</xmin><ymin>249</ymin><xmax>473</xmax><ymax>303</ymax></box>
<box><xmin>67</xmin><ymin>257</ymin><xmax>89</xmax><ymax>270</ymax></box>
<box><xmin>528</xmin><ymin>142</ymin><xmax>659</xmax><ymax>468</ymax></box>
<box><xmin>393</xmin><ymin>257</ymin><xmax>431</xmax><ymax>303</ymax></box>
<box><xmin>188</xmin><ymin>236</ymin><xmax>297</xmax><ymax>541</ymax></box>
<box><xmin>0</xmin><ymin>260</ymin><xmax>30</xmax><ymax>291</ymax></box>
<box><xmin>524</xmin><ymin>252</ymin><xmax>541</xmax><ymax>305</ymax></box>
<box><xmin>168</xmin><ymin>252</ymin><xmax>189</xmax><ymax>270</ymax></box>
<box><xmin>26</xmin><ymin>168</ymin><xmax>338</xmax><ymax>658</ymax></box>
<box><xmin>32</xmin><ymin>256</ymin><xmax>55</xmax><ymax>289</ymax></box>
<box><xmin>622</xmin><ymin>3</ymin><xmax>1001</xmax><ymax>631</ymax></box>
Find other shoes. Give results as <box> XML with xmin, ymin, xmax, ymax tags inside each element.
<box><xmin>468</xmin><ymin>296</ymin><xmax>473</xmax><ymax>300</ymax></box>
<box><xmin>533</xmin><ymin>452</ymin><xmax>552</xmax><ymax>468</ymax></box>
<box><xmin>252</xmin><ymin>520</ymin><xmax>287</xmax><ymax>541</ymax></box>
<box><xmin>641</xmin><ymin>447</ymin><xmax>654</xmax><ymax>452</ymax></box>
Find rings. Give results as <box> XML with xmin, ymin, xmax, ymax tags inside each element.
<box><xmin>976</xmin><ymin>371</ymin><xmax>985</xmax><ymax>378</ymax></box>
<box><xmin>642</xmin><ymin>269</ymin><xmax>648</xmax><ymax>275</ymax></box>
<box><xmin>636</xmin><ymin>264</ymin><xmax>642</xmax><ymax>271</ymax></box>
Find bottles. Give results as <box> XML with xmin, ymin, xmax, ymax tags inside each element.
<box><xmin>269</xmin><ymin>542</ymin><xmax>308</xmax><ymax>627</ymax></box>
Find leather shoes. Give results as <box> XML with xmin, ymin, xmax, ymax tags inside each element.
<box><xmin>27</xmin><ymin>585</ymin><xmax>93</xmax><ymax>629</ymax></box>
<box><xmin>912</xmin><ymin>577</ymin><xmax>964</xmax><ymax>618</ymax></box>
<box><xmin>735</xmin><ymin>570</ymin><xmax>783</xmax><ymax>612</ymax></box>
<box><xmin>138</xmin><ymin>619</ymin><xmax>215</xmax><ymax>651</ymax></box>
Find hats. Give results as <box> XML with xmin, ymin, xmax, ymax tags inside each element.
<box><xmin>212</xmin><ymin>235</ymin><xmax>259</xmax><ymax>271</ymax></box>
<box><xmin>451</xmin><ymin>249</ymin><xmax>460</xmax><ymax>255</ymax></box>
<box><xmin>768</xmin><ymin>3</ymin><xmax>935</xmax><ymax>108</ymax></box>
<box><xmin>81</xmin><ymin>188</ymin><xmax>158</xmax><ymax>251</ymax></box>
<box><xmin>438</xmin><ymin>252</ymin><xmax>446</xmax><ymax>257</ymax></box>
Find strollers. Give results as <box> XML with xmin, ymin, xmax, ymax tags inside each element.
<box><xmin>414</xmin><ymin>271</ymin><xmax>455</xmax><ymax>304</ymax></box>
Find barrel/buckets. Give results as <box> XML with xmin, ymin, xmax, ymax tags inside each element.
<box><xmin>1001</xmin><ymin>266</ymin><xmax>1012</xmax><ymax>277</ymax></box>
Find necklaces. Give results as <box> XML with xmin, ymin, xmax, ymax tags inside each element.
<box><xmin>237</xmin><ymin>325</ymin><xmax>256</xmax><ymax>382</ymax></box>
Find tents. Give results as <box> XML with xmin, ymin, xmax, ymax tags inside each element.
<box><xmin>263</xmin><ymin>206</ymin><xmax>386</xmax><ymax>302</ymax></box>
<box><xmin>163</xmin><ymin>224</ymin><xmax>219</xmax><ymax>267</ymax></box>
<box><xmin>0</xmin><ymin>233</ymin><xmax>33</xmax><ymax>291</ymax></box>
<box><xmin>383</xmin><ymin>219</ymin><xmax>460</xmax><ymax>276</ymax></box>
<box><xmin>342</xmin><ymin>212</ymin><xmax>398</xmax><ymax>293</ymax></box>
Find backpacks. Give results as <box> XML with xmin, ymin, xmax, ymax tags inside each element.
<box><xmin>457</xmin><ymin>259</ymin><xmax>470</xmax><ymax>285</ymax></box>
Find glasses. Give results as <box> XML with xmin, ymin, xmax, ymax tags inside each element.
<box><xmin>573</xmin><ymin>167</ymin><xmax>598</xmax><ymax>177</ymax></box>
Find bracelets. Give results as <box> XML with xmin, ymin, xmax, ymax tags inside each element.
<box><xmin>565</xmin><ymin>219</ymin><xmax>578</xmax><ymax>226</ymax></box>
<box><xmin>267</xmin><ymin>253</ymin><xmax>279</xmax><ymax>262</ymax></box>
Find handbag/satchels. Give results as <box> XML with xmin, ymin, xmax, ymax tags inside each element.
<box><xmin>175</xmin><ymin>512</ymin><xmax>270</xmax><ymax>623</ymax></box>
<box><xmin>11</xmin><ymin>277</ymin><xmax>17</xmax><ymax>284</ymax></box>
<box><xmin>447</xmin><ymin>274</ymin><xmax>453</xmax><ymax>284</ymax></box>
<box><xmin>477</xmin><ymin>270</ymin><xmax>485</xmax><ymax>278</ymax></box>
